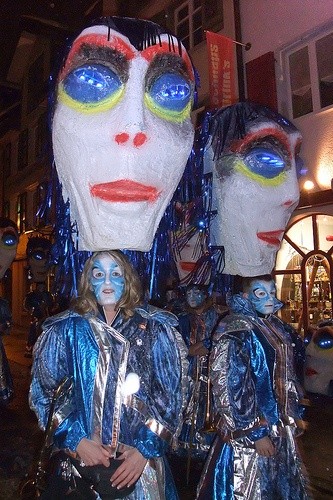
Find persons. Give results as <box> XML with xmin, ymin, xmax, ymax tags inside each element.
<box><xmin>24</xmin><ymin>281</ymin><xmax>52</xmax><ymax>358</ymax></box>
<box><xmin>176</xmin><ymin>285</ymin><xmax>221</xmax><ymax>500</ymax></box>
<box><xmin>26</xmin><ymin>236</ymin><xmax>53</xmax><ymax>282</ymax></box>
<box><xmin>47</xmin><ymin>15</ymin><xmax>206</xmax><ymax>251</ymax></box>
<box><xmin>160</xmin><ymin>289</ymin><xmax>187</xmax><ymax>315</ymax></box>
<box><xmin>169</xmin><ymin>197</ymin><xmax>218</xmax><ymax>285</ymax></box>
<box><xmin>197</xmin><ymin>275</ymin><xmax>313</xmax><ymax>500</ymax></box>
<box><xmin>28</xmin><ymin>249</ymin><xmax>189</xmax><ymax>499</ymax></box>
<box><xmin>0</xmin><ymin>298</ymin><xmax>13</xmax><ymax>405</ymax></box>
<box><xmin>304</xmin><ymin>322</ymin><xmax>333</xmax><ymax>396</ymax></box>
<box><xmin>0</xmin><ymin>218</ymin><xmax>19</xmax><ymax>279</ymax></box>
<box><xmin>193</xmin><ymin>101</ymin><xmax>302</xmax><ymax>278</ymax></box>
